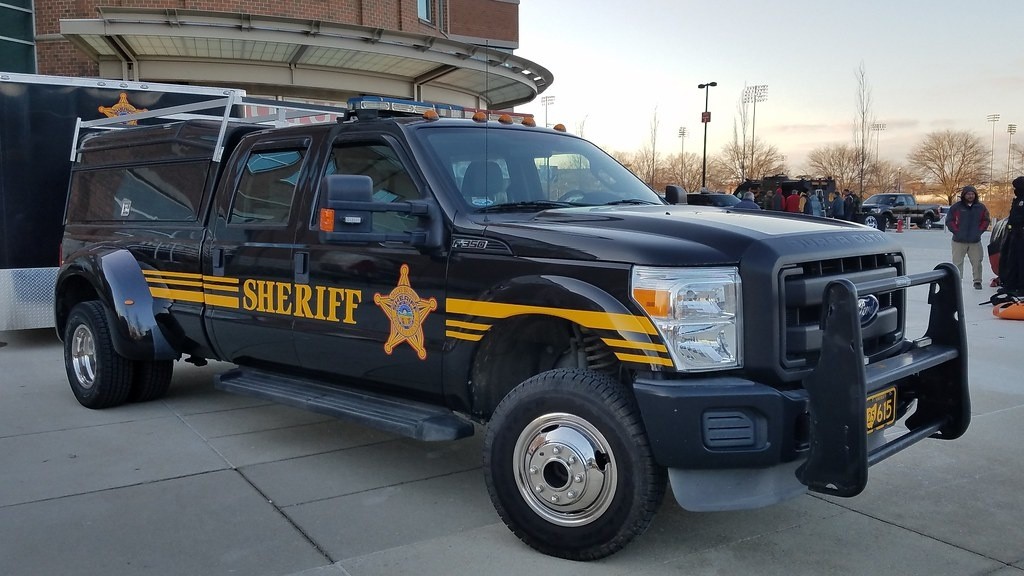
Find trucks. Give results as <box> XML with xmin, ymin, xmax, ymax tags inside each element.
<box><xmin>733</xmin><ymin>175</ymin><xmax>836</xmax><ymax>214</ymax></box>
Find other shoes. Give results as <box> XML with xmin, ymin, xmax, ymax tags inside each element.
<box><xmin>973</xmin><ymin>282</ymin><xmax>982</xmax><ymax>289</ymax></box>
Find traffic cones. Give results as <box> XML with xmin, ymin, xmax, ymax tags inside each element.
<box><xmin>896</xmin><ymin>219</ymin><xmax>903</xmax><ymax>233</ymax></box>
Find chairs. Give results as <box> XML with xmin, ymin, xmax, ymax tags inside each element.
<box><xmin>462</xmin><ymin>160</ymin><xmax>504</xmax><ymax>204</ymax></box>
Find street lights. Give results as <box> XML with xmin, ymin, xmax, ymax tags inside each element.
<box><xmin>898</xmin><ymin>167</ymin><xmax>902</xmax><ymax>193</ymax></box>
<box><xmin>697</xmin><ymin>82</ymin><xmax>717</xmax><ymax>191</ymax></box>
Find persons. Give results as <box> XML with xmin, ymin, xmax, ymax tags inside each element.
<box><xmin>945</xmin><ymin>185</ymin><xmax>991</xmax><ymax>290</ymax></box>
<box><xmin>733</xmin><ymin>188</ymin><xmax>862</xmax><ymax>224</ymax></box>
<box><xmin>996</xmin><ymin>176</ymin><xmax>1024</xmax><ymax>292</ymax></box>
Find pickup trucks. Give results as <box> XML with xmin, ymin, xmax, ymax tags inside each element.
<box><xmin>862</xmin><ymin>193</ymin><xmax>941</xmax><ymax>228</ymax></box>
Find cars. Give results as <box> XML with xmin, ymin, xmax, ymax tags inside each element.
<box><xmin>687</xmin><ymin>194</ymin><xmax>740</xmax><ymax>207</ymax></box>
<box><xmin>931</xmin><ymin>206</ymin><xmax>951</xmax><ymax>227</ymax></box>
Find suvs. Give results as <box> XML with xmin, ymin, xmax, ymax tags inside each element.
<box><xmin>53</xmin><ymin>93</ymin><xmax>970</xmax><ymax>560</ymax></box>
